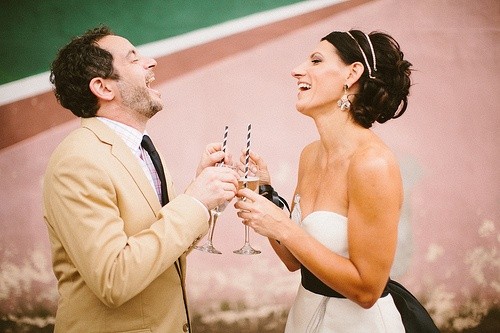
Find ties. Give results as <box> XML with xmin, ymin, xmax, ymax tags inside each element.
<box><xmin>141</xmin><ymin>135</ymin><xmax>180</xmax><ymax>277</ymax></box>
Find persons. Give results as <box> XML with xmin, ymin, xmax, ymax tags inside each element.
<box><xmin>233</xmin><ymin>29</ymin><xmax>444</xmax><ymax>333</ymax></box>
<box><xmin>43</xmin><ymin>24</ymin><xmax>239</xmax><ymax>333</ymax></box>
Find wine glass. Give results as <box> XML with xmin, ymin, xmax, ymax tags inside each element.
<box><xmin>233</xmin><ymin>164</ymin><xmax>259</xmax><ymax>255</ymax></box>
<box><xmin>192</xmin><ymin>163</ymin><xmax>238</xmax><ymax>254</ymax></box>
